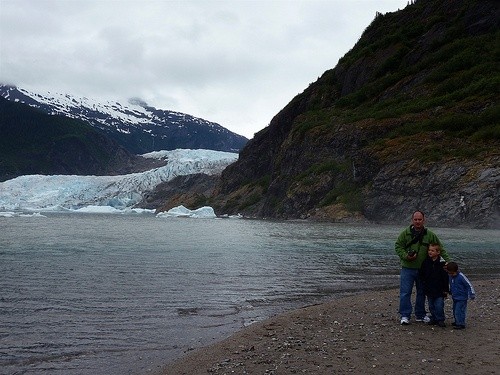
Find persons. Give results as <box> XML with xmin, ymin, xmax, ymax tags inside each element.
<box><xmin>425</xmin><ymin>244</ymin><xmax>449</xmax><ymax>327</ymax></box>
<box><xmin>447</xmin><ymin>261</ymin><xmax>475</xmax><ymax>330</ymax></box>
<box><xmin>395</xmin><ymin>210</ymin><xmax>450</xmax><ymax>325</ymax></box>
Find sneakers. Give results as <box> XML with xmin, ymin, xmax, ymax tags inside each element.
<box><xmin>416</xmin><ymin>319</ymin><xmax>426</xmax><ymax>323</ymax></box>
<box><xmin>400</xmin><ymin>318</ymin><xmax>410</xmax><ymax>325</ymax></box>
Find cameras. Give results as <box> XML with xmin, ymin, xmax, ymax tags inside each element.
<box><xmin>408</xmin><ymin>250</ymin><xmax>416</xmax><ymax>256</ymax></box>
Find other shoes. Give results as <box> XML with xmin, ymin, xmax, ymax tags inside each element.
<box><xmin>439</xmin><ymin>322</ymin><xmax>446</xmax><ymax>327</ymax></box>
<box><xmin>428</xmin><ymin>322</ymin><xmax>438</xmax><ymax>325</ymax></box>
<box><xmin>453</xmin><ymin>325</ymin><xmax>465</xmax><ymax>329</ymax></box>
<box><xmin>451</xmin><ymin>322</ymin><xmax>456</xmax><ymax>325</ymax></box>
